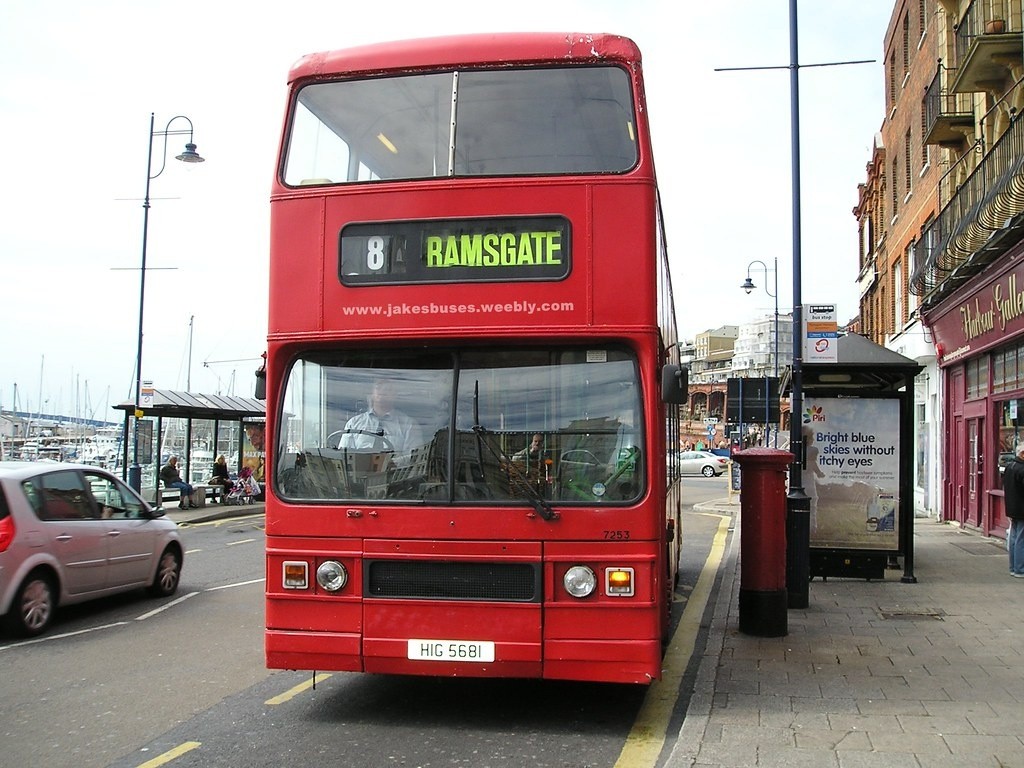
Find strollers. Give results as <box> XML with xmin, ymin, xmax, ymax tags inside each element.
<box><xmin>225</xmin><ymin>464</ymin><xmax>257</xmax><ymax>505</ymax></box>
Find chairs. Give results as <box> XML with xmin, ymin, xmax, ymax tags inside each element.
<box><xmin>44</xmin><ymin>475</ymin><xmax>84</xmax><ymax>519</ymax></box>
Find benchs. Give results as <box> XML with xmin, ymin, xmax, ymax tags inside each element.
<box><xmin>158</xmin><ymin>481</ymin><xmax>264</xmax><ymax>507</ymax></box>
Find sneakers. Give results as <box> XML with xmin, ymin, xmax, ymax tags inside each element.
<box><xmin>189</xmin><ymin>504</ymin><xmax>199</xmax><ymax>508</ymax></box>
<box><xmin>178</xmin><ymin>504</ymin><xmax>188</xmax><ymax>510</ymax></box>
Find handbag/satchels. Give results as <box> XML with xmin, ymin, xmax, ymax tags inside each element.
<box><xmin>208</xmin><ymin>476</ymin><xmax>221</xmax><ymax>485</ymax></box>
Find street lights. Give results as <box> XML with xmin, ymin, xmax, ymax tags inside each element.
<box><xmin>740</xmin><ymin>257</ymin><xmax>779</xmax><ymax>449</ymax></box>
<box><xmin>129</xmin><ymin>112</ymin><xmax>206</xmax><ymax>496</ymax></box>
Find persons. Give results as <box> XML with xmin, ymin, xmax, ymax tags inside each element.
<box><xmin>802</xmin><ymin>426</ymin><xmax>825</xmax><ymax>543</ymax></box>
<box><xmin>335</xmin><ymin>379</ymin><xmax>422</xmax><ymax>482</ymax></box>
<box><xmin>1003</xmin><ymin>442</ymin><xmax>1024</xmax><ymax>578</ymax></box>
<box><xmin>512</xmin><ymin>434</ymin><xmax>544</xmax><ymax>461</ymax></box>
<box><xmin>88</xmin><ymin>481</ymin><xmax>113</xmax><ymax>518</ymax></box>
<box><xmin>717</xmin><ymin>429</ymin><xmax>762</xmax><ymax>448</ymax></box>
<box><xmin>212</xmin><ymin>454</ymin><xmax>235</xmax><ymax>501</ymax></box>
<box><xmin>158</xmin><ymin>456</ymin><xmax>199</xmax><ymax>510</ymax></box>
<box><xmin>244</xmin><ymin>424</ymin><xmax>265</xmax><ymax>475</ymax></box>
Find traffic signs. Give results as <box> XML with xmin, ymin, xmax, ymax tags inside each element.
<box><xmin>617</xmin><ymin>447</ymin><xmax>636</xmax><ymax>472</ymax></box>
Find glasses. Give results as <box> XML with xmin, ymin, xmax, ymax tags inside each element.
<box><xmin>373</xmin><ymin>383</ymin><xmax>394</xmax><ymax>391</ymax></box>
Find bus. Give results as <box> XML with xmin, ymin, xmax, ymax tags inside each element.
<box><xmin>266</xmin><ymin>34</ymin><xmax>689</xmax><ymax>688</ymax></box>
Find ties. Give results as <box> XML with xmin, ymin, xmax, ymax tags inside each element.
<box><xmin>373</xmin><ymin>416</ymin><xmax>384</xmax><ymax>450</ymax></box>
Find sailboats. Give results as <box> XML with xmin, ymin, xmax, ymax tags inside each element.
<box><xmin>0</xmin><ymin>314</ymin><xmax>237</xmax><ymax>488</ymax></box>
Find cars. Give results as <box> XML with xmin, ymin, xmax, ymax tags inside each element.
<box><xmin>0</xmin><ymin>461</ymin><xmax>186</xmax><ymax>639</ymax></box>
<box><xmin>561</xmin><ymin>449</ymin><xmax>613</xmax><ymax>470</ymax></box>
<box><xmin>680</xmin><ymin>450</ymin><xmax>730</xmax><ymax>478</ymax></box>
<box><xmin>90</xmin><ymin>477</ymin><xmax>157</xmax><ymax>506</ymax></box>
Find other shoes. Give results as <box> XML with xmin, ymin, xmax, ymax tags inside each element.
<box><xmin>1010</xmin><ymin>572</ymin><xmax>1024</xmax><ymax>578</ymax></box>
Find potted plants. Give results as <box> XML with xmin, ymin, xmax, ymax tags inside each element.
<box><xmin>986</xmin><ymin>15</ymin><xmax>1005</xmax><ymax>33</ymax></box>
